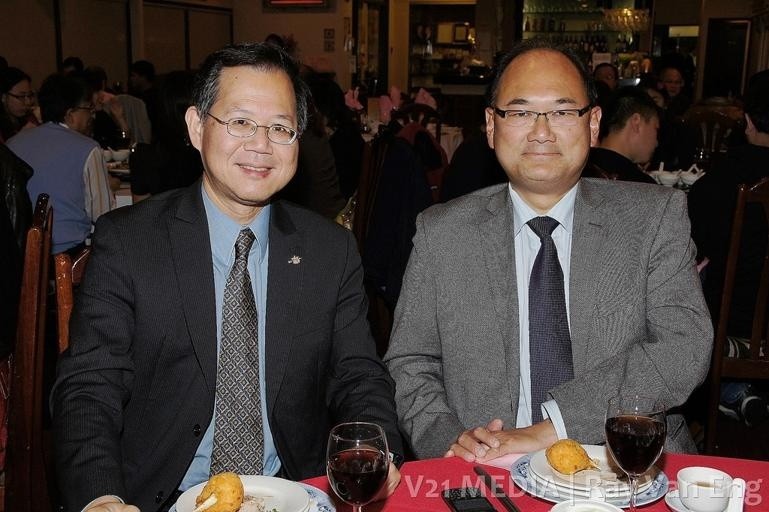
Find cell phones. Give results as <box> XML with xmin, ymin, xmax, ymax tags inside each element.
<box><xmin>441</xmin><ymin>487</ymin><xmax>498</xmax><ymax>512</ymax></box>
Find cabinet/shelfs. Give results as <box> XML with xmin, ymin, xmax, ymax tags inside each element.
<box><xmin>522</xmin><ymin>1</ymin><xmax>635</xmax><ymax>59</ymax></box>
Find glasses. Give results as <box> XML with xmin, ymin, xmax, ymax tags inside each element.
<box><xmin>201</xmin><ymin>109</ymin><xmax>299</xmax><ymax>146</ymax></box>
<box><xmin>492</xmin><ymin>104</ymin><xmax>594</xmax><ymax>128</ymax></box>
<box><xmin>6</xmin><ymin>92</ymin><xmax>35</xmax><ymax>102</ymax></box>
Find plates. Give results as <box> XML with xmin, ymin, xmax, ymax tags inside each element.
<box><xmin>664</xmin><ymin>478</ymin><xmax>749</xmax><ymax>512</ymax></box>
<box><xmin>167</xmin><ymin>475</ymin><xmax>337</xmax><ymax>512</ymax></box>
<box><xmin>108</xmin><ymin>166</ymin><xmax>131</xmax><ymax>176</ymax></box>
<box><xmin>510</xmin><ymin>444</ymin><xmax>670</xmax><ymax>512</ymax></box>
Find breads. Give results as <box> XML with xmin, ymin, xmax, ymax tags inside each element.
<box><xmin>195</xmin><ymin>471</ymin><xmax>245</xmax><ymax>510</ymax></box>
<box><xmin>545</xmin><ymin>437</ymin><xmax>597</xmax><ymax>475</ymax></box>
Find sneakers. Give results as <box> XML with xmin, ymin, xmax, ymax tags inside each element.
<box><xmin>717</xmin><ymin>395</ymin><xmax>767</xmax><ymax>431</ymax></box>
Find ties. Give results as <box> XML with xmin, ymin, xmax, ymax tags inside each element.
<box><xmin>207</xmin><ymin>228</ymin><xmax>269</xmax><ymax>478</ymax></box>
<box><xmin>522</xmin><ymin>217</ymin><xmax>578</xmax><ymax>427</ymax></box>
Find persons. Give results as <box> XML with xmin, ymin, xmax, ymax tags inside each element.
<box><xmin>384</xmin><ymin>37</ymin><xmax>714</xmax><ymax>459</ymax></box>
<box><xmin>44</xmin><ymin>40</ymin><xmax>407</xmax><ymax>512</ymax></box>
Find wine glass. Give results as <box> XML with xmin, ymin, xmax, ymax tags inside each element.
<box><xmin>604</xmin><ymin>395</ymin><xmax>667</xmax><ymax>512</ymax></box>
<box><xmin>326</xmin><ymin>423</ymin><xmax>390</xmax><ymax>512</ymax></box>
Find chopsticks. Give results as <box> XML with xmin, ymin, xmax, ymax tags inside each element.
<box><xmin>473</xmin><ymin>465</ymin><xmax>523</xmax><ymax>512</ymax></box>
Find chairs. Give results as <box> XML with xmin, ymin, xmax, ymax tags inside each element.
<box><xmin>56</xmin><ymin>249</ymin><xmax>95</xmax><ymax>359</ymax></box>
<box><xmin>348</xmin><ymin>137</ymin><xmax>393</xmax><ymax>261</ymax></box>
<box><xmin>3</xmin><ymin>191</ymin><xmax>57</xmax><ymax>511</ymax></box>
<box><xmin>389</xmin><ymin>103</ymin><xmax>441</xmax><ymax>147</ymax></box>
<box><xmin>694</xmin><ymin>111</ymin><xmax>745</xmax><ymax>177</ymax></box>
<box><xmin>697</xmin><ymin>179</ymin><xmax>769</xmax><ymax>455</ymax></box>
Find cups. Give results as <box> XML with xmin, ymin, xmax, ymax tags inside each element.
<box><xmin>676</xmin><ymin>465</ymin><xmax>731</xmax><ymax>512</ymax></box>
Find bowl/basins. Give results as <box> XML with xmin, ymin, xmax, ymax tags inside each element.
<box><xmin>101</xmin><ymin>150</ymin><xmax>131</xmax><ymax>162</ymax></box>
<box><xmin>648</xmin><ymin>171</ymin><xmax>700</xmax><ymax>188</ymax></box>
